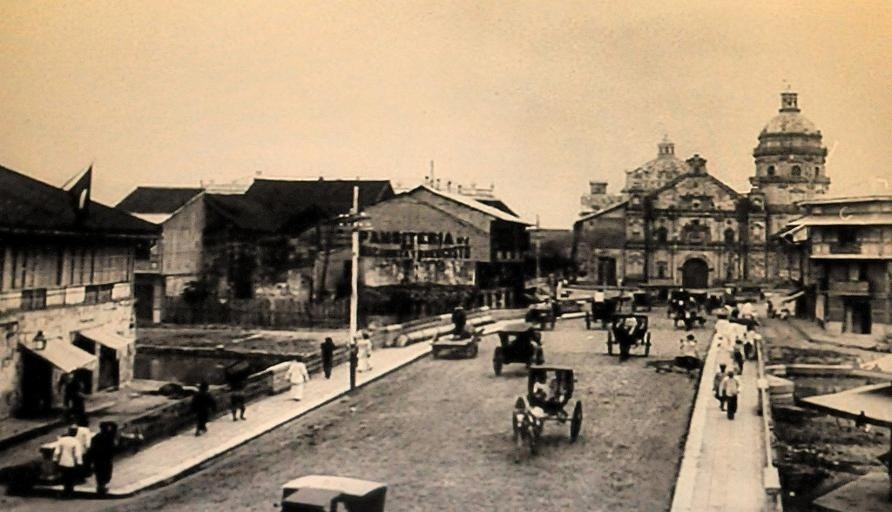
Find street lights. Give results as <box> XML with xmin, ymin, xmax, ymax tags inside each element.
<box><xmin>337</xmin><ymin>206</ymin><xmax>374</xmax><ymax>396</ymax></box>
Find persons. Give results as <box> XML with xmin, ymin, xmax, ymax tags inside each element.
<box><xmin>230</xmin><ymin>379</ymin><xmax>246</xmax><ymax>422</ymax></box>
<box><xmin>666</xmin><ymin>279</ymin><xmax>790</xmax><ymax>419</ymax></box>
<box><xmin>321</xmin><ymin>332</ymin><xmax>372</xmax><ymax>378</ymax></box>
<box><xmin>52</xmin><ymin>373</ymin><xmax>118</xmax><ymax>498</ymax></box>
<box><xmin>452</xmin><ymin>273</ymin><xmax>645</xmax><ymax>369</ymax></box>
<box><xmin>533</xmin><ymin>369</ymin><xmax>568</xmax><ymax>405</ymax></box>
<box><xmin>189</xmin><ymin>379</ymin><xmax>218</xmax><ymax>436</ymax></box>
<box><xmin>285</xmin><ymin>356</ymin><xmax>309</xmax><ymax>401</ymax></box>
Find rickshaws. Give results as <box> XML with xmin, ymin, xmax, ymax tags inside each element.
<box><xmin>583</xmin><ymin>288</ymin><xmax>728</xmax><ymax>363</ymax></box>
<box><xmin>430</xmin><ymin>327</ymin><xmax>485</xmax><ymax>359</ymax></box>
<box><xmin>513</xmin><ymin>367</ymin><xmax>583</xmax><ymax>453</ymax></box>
<box><xmin>493</xmin><ymin>323</ymin><xmax>544</xmax><ymax>376</ymax></box>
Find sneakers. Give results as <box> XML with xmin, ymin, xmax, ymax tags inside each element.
<box><xmin>194</xmin><ymin>426</ymin><xmax>207</xmax><ymax>437</ymax></box>
<box><xmin>232</xmin><ymin>416</ymin><xmax>246</xmax><ymax>421</ymax></box>
